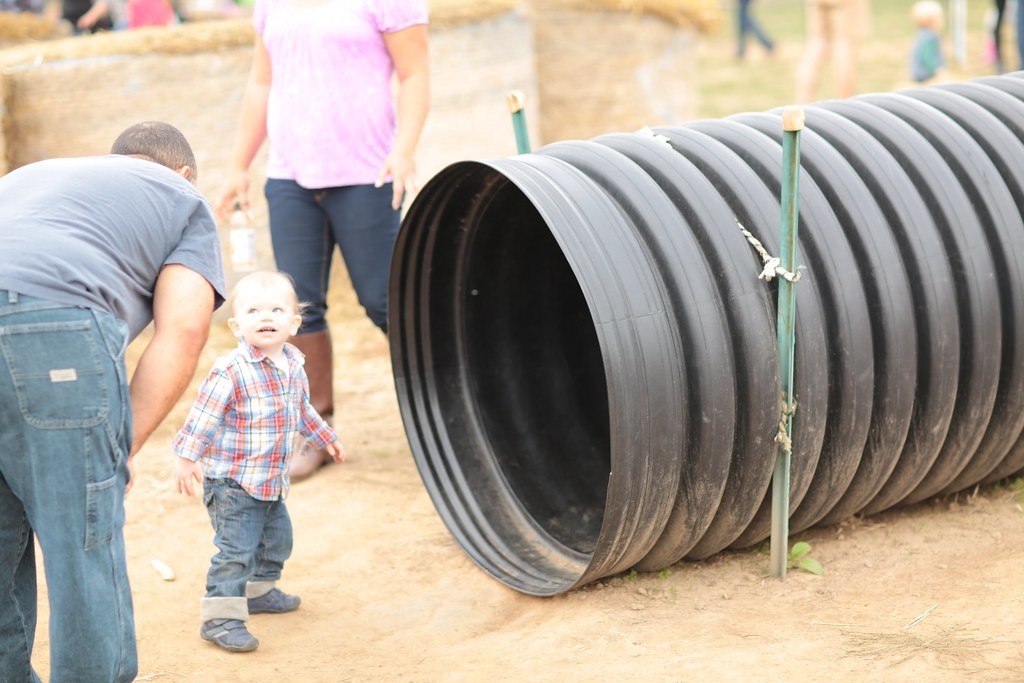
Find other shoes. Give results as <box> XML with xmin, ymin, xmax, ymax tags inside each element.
<box><xmin>288</xmin><ymin>407</ymin><xmax>334</xmax><ymax>480</ymax></box>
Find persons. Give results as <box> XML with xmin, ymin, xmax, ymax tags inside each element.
<box><xmin>731</xmin><ymin>0</ymin><xmax>1024</xmax><ymax>106</ymax></box>
<box><xmin>0</xmin><ymin>121</ymin><xmax>228</xmax><ymax>683</ymax></box>
<box><xmin>216</xmin><ymin>0</ymin><xmax>431</xmax><ymax>485</ymax></box>
<box><xmin>44</xmin><ymin>0</ymin><xmax>182</xmax><ymax>36</ymax></box>
<box><xmin>169</xmin><ymin>270</ymin><xmax>346</xmax><ymax>653</ymax></box>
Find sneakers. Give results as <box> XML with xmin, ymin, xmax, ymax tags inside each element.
<box><xmin>200</xmin><ymin>619</ymin><xmax>259</xmax><ymax>651</ymax></box>
<box><xmin>247</xmin><ymin>587</ymin><xmax>301</xmax><ymax>614</ymax></box>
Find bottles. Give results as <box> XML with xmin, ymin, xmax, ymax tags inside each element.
<box><xmin>228</xmin><ymin>203</ymin><xmax>257</xmax><ymax>273</ymax></box>
